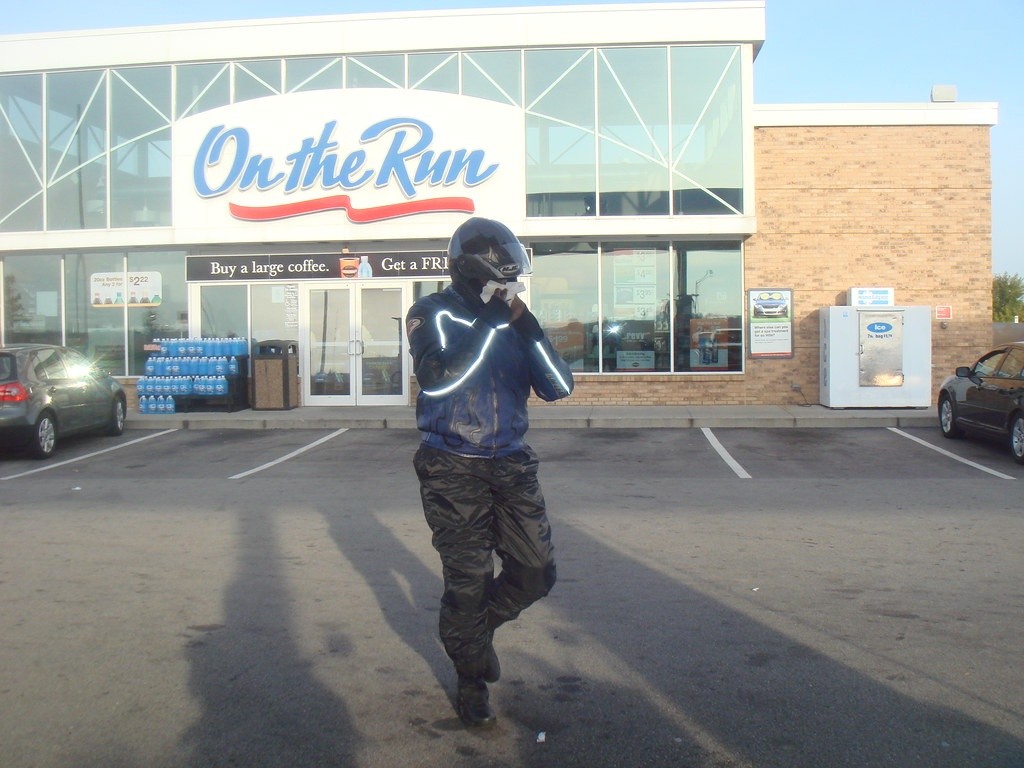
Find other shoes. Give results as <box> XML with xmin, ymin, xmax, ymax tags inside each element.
<box><xmin>456</xmin><ymin>675</ymin><xmax>495</xmax><ymax>727</ymax></box>
<box><xmin>481</xmin><ymin>644</ymin><xmax>500</xmax><ymax>683</ymax></box>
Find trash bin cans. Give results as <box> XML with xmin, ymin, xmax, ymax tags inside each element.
<box><xmin>252</xmin><ymin>339</ymin><xmax>300</xmax><ymax>411</ymax></box>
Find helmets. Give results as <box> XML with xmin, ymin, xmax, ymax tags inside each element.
<box><xmin>447</xmin><ymin>217</ymin><xmax>534</xmax><ymax>308</ymax></box>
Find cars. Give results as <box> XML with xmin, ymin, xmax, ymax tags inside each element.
<box><xmin>938</xmin><ymin>341</ymin><xmax>1024</xmax><ymax>465</ymax></box>
<box><xmin>0</xmin><ymin>343</ymin><xmax>128</xmax><ymax>459</ymax></box>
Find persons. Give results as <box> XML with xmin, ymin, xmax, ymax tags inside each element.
<box><xmin>405</xmin><ymin>216</ymin><xmax>575</xmax><ymax>731</ymax></box>
